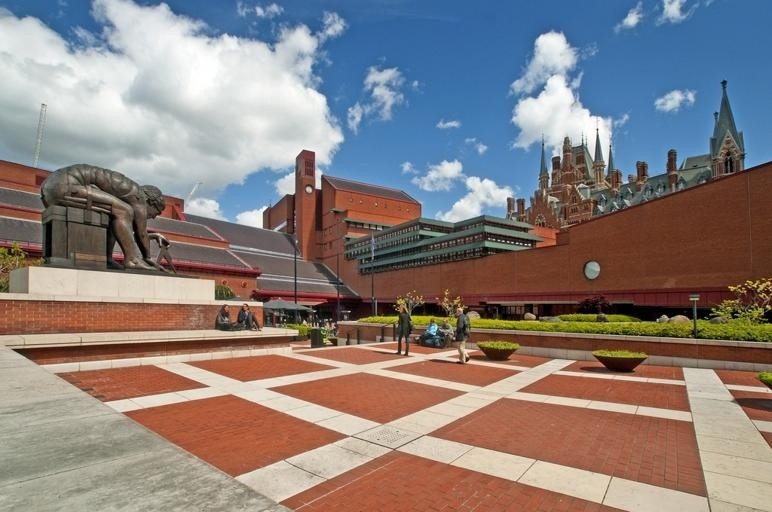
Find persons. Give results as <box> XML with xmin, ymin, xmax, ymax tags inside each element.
<box><xmin>239</xmin><ymin>304</ymin><xmax>264</xmax><ymax>331</ymax></box>
<box><xmin>395</xmin><ymin>305</ymin><xmax>411</xmax><ymax>356</ymax></box>
<box><xmin>454</xmin><ymin>309</ymin><xmax>469</xmax><ymax>362</ymax></box>
<box><xmin>414</xmin><ymin>319</ymin><xmax>437</xmax><ymax>343</ymax></box>
<box><xmin>40</xmin><ymin>163</ymin><xmax>171</xmax><ymax>270</ymax></box>
<box><xmin>440</xmin><ymin>318</ymin><xmax>452</xmax><ymax>347</ymax></box>
<box><xmin>216</xmin><ymin>305</ymin><xmax>241</xmax><ymax>331</ymax></box>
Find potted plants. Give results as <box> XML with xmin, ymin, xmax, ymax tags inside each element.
<box><xmin>476</xmin><ymin>339</ymin><xmax>521</xmax><ymax>360</ymax></box>
<box><xmin>593</xmin><ymin>344</ymin><xmax>649</xmax><ymax>372</ymax></box>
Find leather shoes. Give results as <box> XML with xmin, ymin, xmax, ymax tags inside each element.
<box><xmin>466</xmin><ymin>356</ymin><xmax>470</xmax><ymax>362</ymax></box>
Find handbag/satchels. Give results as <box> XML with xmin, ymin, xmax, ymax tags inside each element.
<box><xmin>407</xmin><ymin>323</ymin><xmax>414</xmax><ymax>332</ymax></box>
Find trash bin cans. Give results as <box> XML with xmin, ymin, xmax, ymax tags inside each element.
<box><xmin>311</xmin><ymin>329</ymin><xmax>321</xmax><ymax>348</ymax></box>
